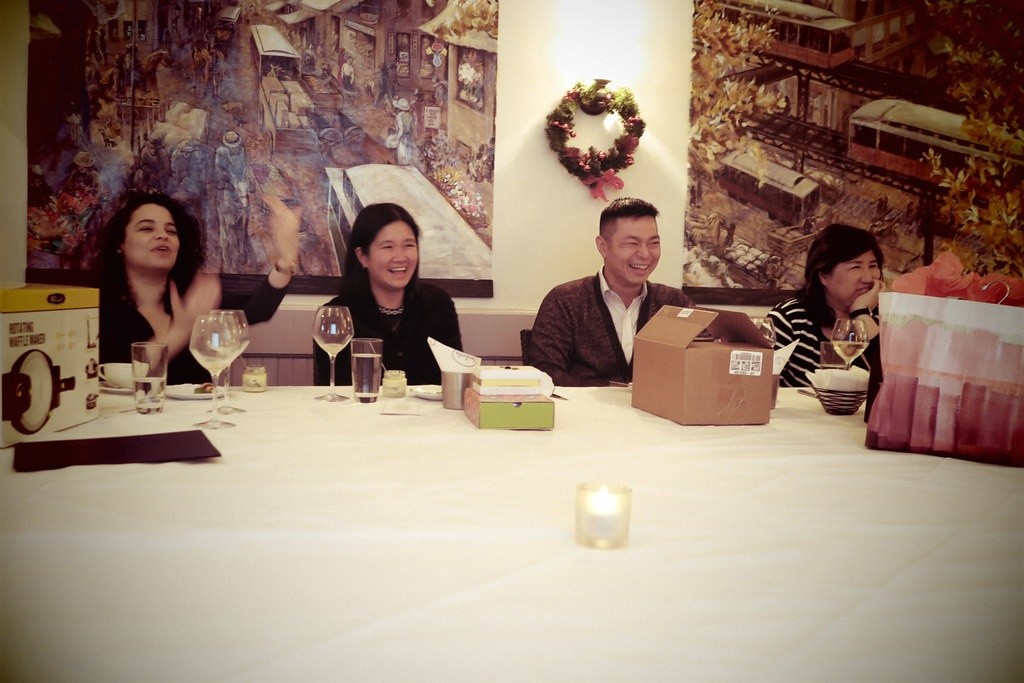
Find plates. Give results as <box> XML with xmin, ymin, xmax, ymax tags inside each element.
<box><xmin>165</xmin><ymin>384</ymin><xmax>224</xmax><ymax>400</ymax></box>
<box><xmin>409</xmin><ymin>385</ymin><xmax>442</xmax><ymax>401</ymax></box>
<box><xmin>97</xmin><ymin>381</ymin><xmax>134</xmax><ymax>396</ymax></box>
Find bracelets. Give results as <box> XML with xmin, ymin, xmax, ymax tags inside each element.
<box><xmin>274</xmin><ymin>262</ymin><xmax>297</xmax><ymax>275</ymax></box>
<box><xmin>850</xmin><ymin>307</ymin><xmax>873</xmax><ymax>318</ymax></box>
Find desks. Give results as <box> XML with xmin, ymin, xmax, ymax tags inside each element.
<box><xmin>0</xmin><ymin>382</ymin><xmax>1024</xmax><ymax>681</ymax></box>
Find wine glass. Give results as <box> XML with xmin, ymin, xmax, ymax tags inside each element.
<box><xmin>209</xmin><ymin>309</ymin><xmax>251</xmax><ymax>415</ymax></box>
<box><xmin>313</xmin><ymin>305</ymin><xmax>354</xmax><ymax>401</ymax></box>
<box><xmin>831</xmin><ymin>317</ymin><xmax>869</xmax><ymax>371</ymax></box>
<box><xmin>190</xmin><ymin>314</ymin><xmax>240</xmax><ymax>429</ymax></box>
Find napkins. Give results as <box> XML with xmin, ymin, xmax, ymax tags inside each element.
<box><xmin>805</xmin><ymin>365</ymin><xmax>871</xmax><ymax>411</ymax></box>
<box><xmin>773</xmin><ymin>337</ymin><xmax>800</xmax><ymax>374</ymax></box>
<box><xmin>427</xmin><ymin>337</ymin><xmax>481</xmax><ymax>373</ymax></box>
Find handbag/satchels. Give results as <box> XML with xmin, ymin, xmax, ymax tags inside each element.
<box><xmin>864</xmin><ymin>289</ymin><xmax>1023</xmax><ymax>467</ymax></box>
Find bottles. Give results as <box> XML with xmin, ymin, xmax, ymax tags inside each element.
<box><xmin>242</xmin><ymin>365</ymin><xmax>267</xmax><ymax>391</ymax></box>
<box><xmin>381</xmin><ymin>370</ymin><xmax>406</xmax><ymax>398</ymax></box>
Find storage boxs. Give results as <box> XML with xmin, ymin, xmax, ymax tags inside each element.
<box><xmin>632</xmin><ymin>305</ymin><xmax>772</xmax><ymax>426</ymax></box>
<box><xmin>0</xmin><ymin>281</ymin><xmax>100</xmax><ymax>448</ymax></box>
<box><xmin>465</xmin><ymin>365</ymin><xmax>555</xmax><ymax>432</ymax></box>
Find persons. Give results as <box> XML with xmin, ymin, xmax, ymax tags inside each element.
<box><xmin>85</xmin><ymin>190</ymin><xmax>304</xmax><ymax>385</ymax></box>
<box><xmin>527</xmin><ymin>198</ymin><xmax>699</xmax><ymax>387</ymax></box>
<box><xmin>313</xmin><ymin>202</ymin><xmax>464</xmax><ymax>386</ymax></box>
<box><xmin>759</xmin><ymin>224</ymin><xmax>887</xmax><ymax>388</ymax></box>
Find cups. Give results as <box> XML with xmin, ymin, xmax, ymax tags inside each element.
<box><xmin>131</xmin><ymin>342</ymin><xmax>169</xmax><ymax>415</ymax></box>
<box><xmin>819</xmin><ymin>342</ymin><xmax>846</xmax><ymax>370</ymax></box>
<box><xmin>441</xmin><ymin>371</ymin><xmax>473</xmax><ymax>410</ymax></box>
<box><xmin>351</xmin><ymin>337</ymin><xmax>383</xmax><ymax>403</ymax></box>
<box><xmin>98</xmin><ymin>363</ymin><xmax>149</xmax><ymax>387</ymax></box>
